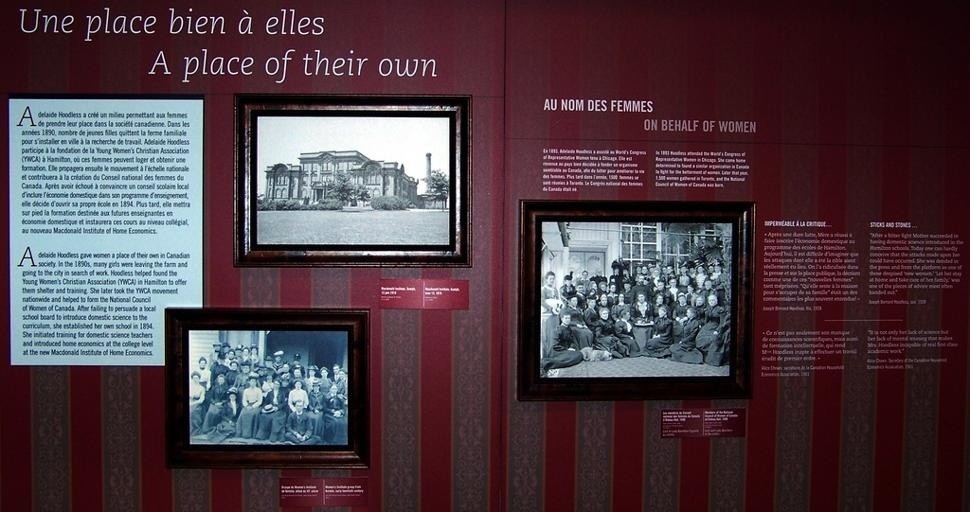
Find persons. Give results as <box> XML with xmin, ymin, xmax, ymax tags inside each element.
<box><xmin>187</xmin><ymin>343</ymin><xmax>350</xmax><ymax>447</ymax></box>
<box><xmin>541</xmin><ymin>260</ymin><xmax>731</xmax><ymax>372</ymax></box>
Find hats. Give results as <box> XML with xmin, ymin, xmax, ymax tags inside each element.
<box><xmin>241</xmin><ymin>361</ymin><xmax>251</xmax><ymax>367</ymax></box>
<box><xmin>264</xmin><ymin>356</ymin><xmax>272</xmax><ymax>361</ymax></box>
<box><xmin>308</xmin><ymin>366</ymin><xmax>315</xmax><ymax>370</ymax></box>
<box><xmin>295</xmin><ymin>352</ymin><xmax>301</xmax><ymax>356</ymax></box>
<box><xmin>261</xmin><ymin>404</ymin><xmax>275</xmax><ymax>414</ymax></box>
<box><xmin>222</xmin><ymin>342</ymin><xmax>232</xmax><ymax>348</ymax></box>
<box><xmin>273</xmin><ymin>350</ymin><xmax>285</xmax><ymax>356</ymax></box>
<box><xmin>312</xmin><ymin>382</ymin><xmax>320</xmax><ymax>387</ymax></box>
<box><xmin>235</xmin><ymin>344</ymin><xmax>243</xmax><ymax>350</ymax></box>
<box><xmin>230</xmin><ymin>361</ymin><xmax>239</xmax><ymax>366</ymax></box>
<box><xmin>213</xmin><ymin>342</ymin><xmax>222</xmax><ymax>346</ymax></box>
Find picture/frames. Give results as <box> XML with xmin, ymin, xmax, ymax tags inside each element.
<box><xmin>519</xmin><ymin>200</ymin><xmax>754</xmax><ymax>402</ymax></box>
<box><xmin>234</xmin><ymin>92</ymin><xmax>474</xmax><ymax>267</ymax></box>
<box><xmin>164</xmin><ymin>307</ymin><xmax>372</xmax><ymax>470</ymax></box>
